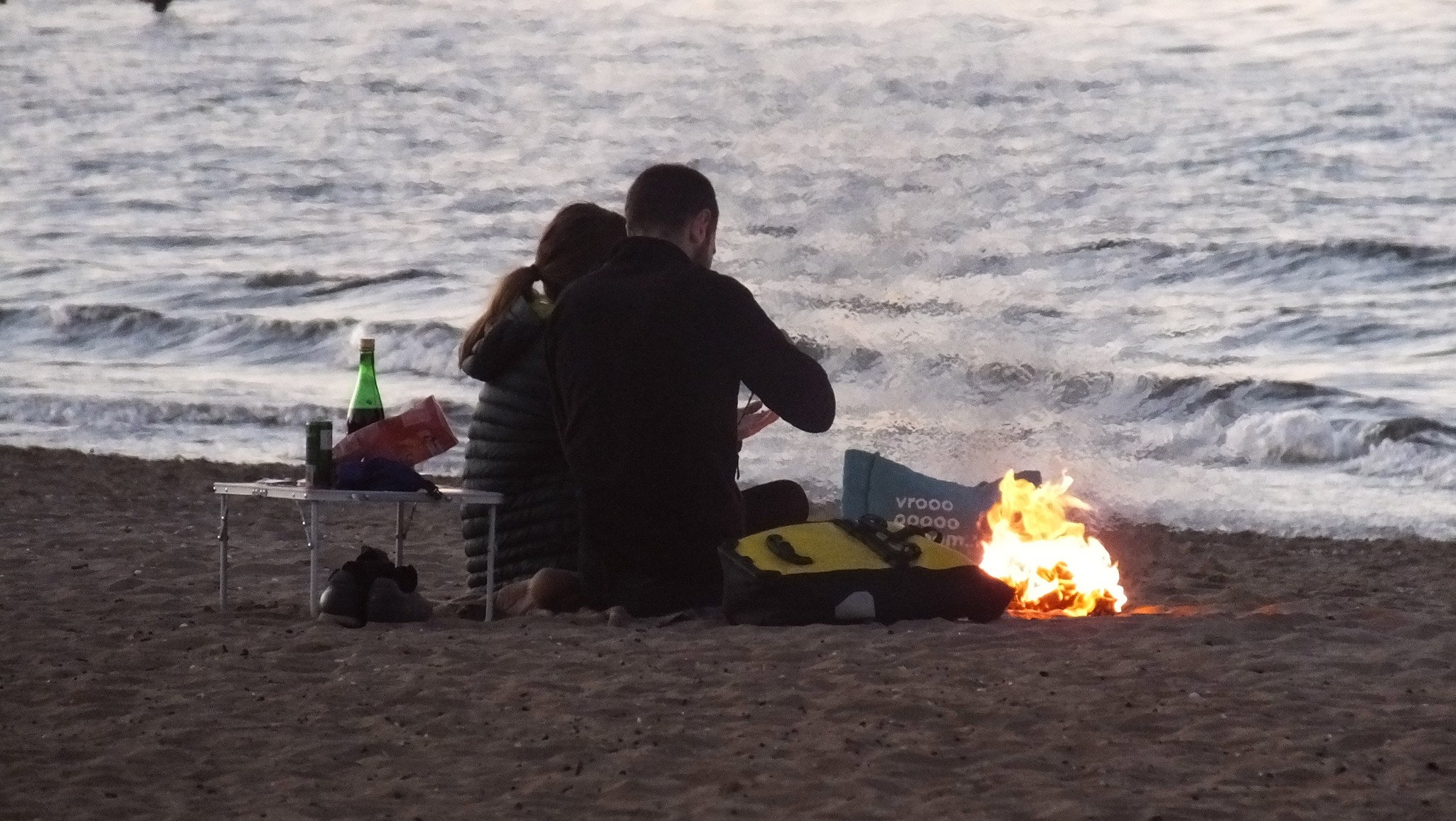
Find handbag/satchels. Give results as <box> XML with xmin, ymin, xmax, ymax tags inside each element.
<box><xmin>841</xmin><ymin>449</ymin><xmax>1038</xmax><ymax>561</ymax></box>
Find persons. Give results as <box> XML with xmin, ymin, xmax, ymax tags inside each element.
<box><xmin>513</xmin><ymin>163</ymin><xmax>836</xmax><ymax>622</ymax></box>
<box><xmin>458</xmin><ymin>202</ymin><xmax>629</xmax><ymax>596</ymax></box>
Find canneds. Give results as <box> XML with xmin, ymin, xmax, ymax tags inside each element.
<box><xmin>305</xmin><ymin>420</ymin><xmax>334</xmax><ymax>489</ymax></box>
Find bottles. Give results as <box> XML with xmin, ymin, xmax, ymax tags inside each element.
<box><xmin>347</xmin><ymin>338</ymin><xmax>385</xmax><ymax>434</ymax></box>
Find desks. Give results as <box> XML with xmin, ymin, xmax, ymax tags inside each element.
<box><xmin>213</xmin><ymin>482</ymin><xmax>504</xmax><ymax>623</ymax></box>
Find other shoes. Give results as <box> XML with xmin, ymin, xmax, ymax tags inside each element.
<box><xmin>317</xmin><ymin>545</ymin><xmax>433</xmax><ymax>629</ymax></box>
